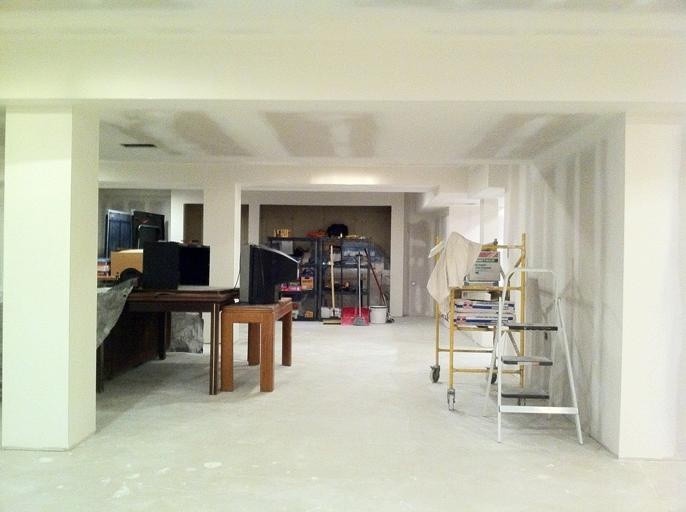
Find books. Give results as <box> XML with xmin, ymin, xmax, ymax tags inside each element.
<box><xmin>467</xmin><ymin>249</ymin><xmax>501</xmax><ymax>282</ymax></box>
<box><xmin>439</xmin><ymin>290</ymin><xmax>516</xmax><ymax>327</ymax></box>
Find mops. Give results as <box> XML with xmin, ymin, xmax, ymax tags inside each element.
<box><xmin>363</xmin><ymin>248</ymin><xmax>396</xmax><ymax>324</ymax></box>
<box><xmin>352</xmin><ymin>249</ymin><xmax>366</xmax><ymax>326</ymax></box>
<box><xmin>321</xmin><ymin>245</ymin><xmax>341</xmax><ymax>324</ymax></box>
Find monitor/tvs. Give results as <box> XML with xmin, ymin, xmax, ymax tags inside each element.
<box><xmin>239</xmin><ymin>244</ymin><xmax>299</xmax><ymax>304</ymax></box>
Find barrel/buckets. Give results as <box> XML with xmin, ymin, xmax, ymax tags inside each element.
<box><xmin>368</xmin><ymin>305</ymin><xmax>389</xmax><ymax>324</ymax></box>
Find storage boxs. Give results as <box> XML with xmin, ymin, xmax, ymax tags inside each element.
<box><xmin>109</xmin><ymin>247</ymin><xmax>145</xmax><ymax>279</ymax></box>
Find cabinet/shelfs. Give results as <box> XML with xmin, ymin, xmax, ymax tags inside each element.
<box><xmin>318</xmin><ymin>237</ymin><xmax>372</xmax><ymax>323</ymax></box>
<box><xmin>427</xmin><ymin>232</ymin><xmax>527</xmax><ymax>412</ymax></box>
<box><xmin>269</xmin><ymin>237</ymin><xmax>317</xmax><ymax>321</ymax></box>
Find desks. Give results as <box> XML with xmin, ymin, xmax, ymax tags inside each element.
<box><xmin>94</xmin><ymin>283</ymin><xmax>238</xmax><ymax>395</ymax></box>
<box><xmin>218</xmin><ymin>294</ymin><xmax>295</xmax><ymax>394</ymax></box>
